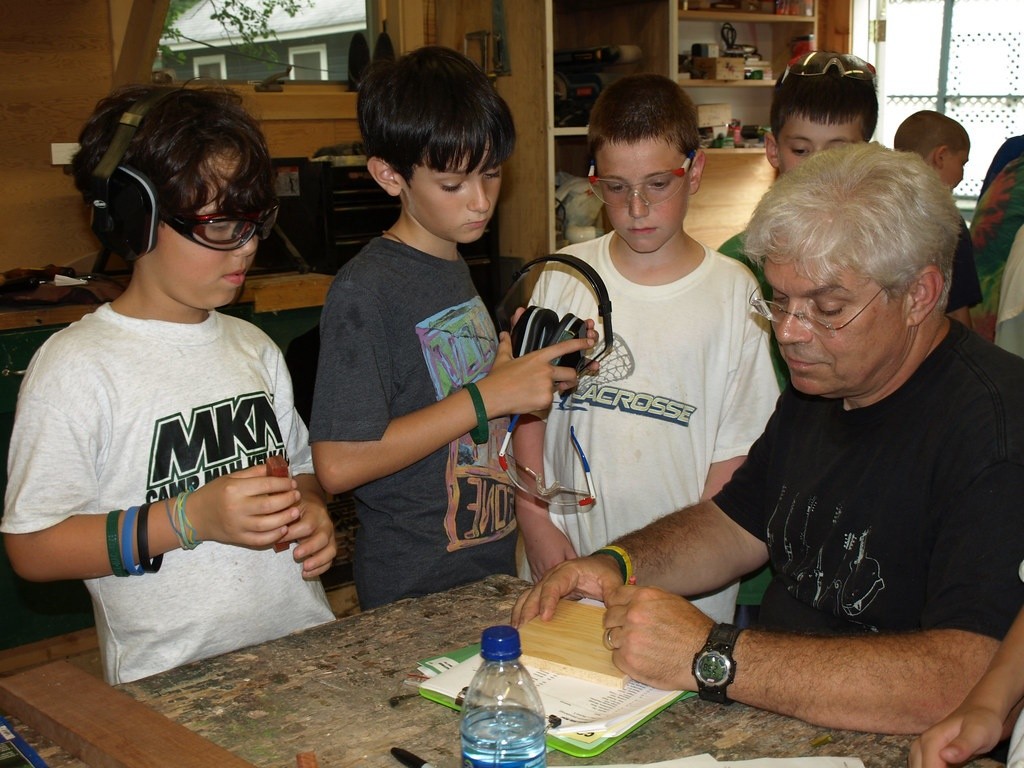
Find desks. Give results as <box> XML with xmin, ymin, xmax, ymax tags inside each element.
<box><xmin>0</xmin><ymin>577</ymin><xmax>1009</xmax><ymax>768</ymax></box>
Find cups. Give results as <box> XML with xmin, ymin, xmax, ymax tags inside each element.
<box><xmin>789</xmin><ymin>34</ymin><xmax>815</xmax><ymax>56</ymax></box>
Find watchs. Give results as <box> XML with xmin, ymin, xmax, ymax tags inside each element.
<box><xmin>691</xmin><ymin>623</ymin><xmax>743</xmax><ymax>706</ymax></box>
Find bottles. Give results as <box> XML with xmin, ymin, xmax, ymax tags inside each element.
<box><xmin>459</xmin><ymin>625</ymin><xmax>548</xmax><ymax>768</ymax></box>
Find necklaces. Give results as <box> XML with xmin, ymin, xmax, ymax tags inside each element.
<box><xmin>382</xmin><ymin>231</ymin><xmax>404</xmax><ymax>243</ymax></box>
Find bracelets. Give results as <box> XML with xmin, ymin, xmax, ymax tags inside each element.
<box><xmin>106</xmin><ymin>510</ymin><xmax>130</xmax><ymax>577</ymax></box>
<box><xmin>164</xmin><ymin>486</ymin><xmax>202</xmax><ymax>550</ymax></box>
<box><xmin>137</xmin><ymin>503</ymin><xmax>163</xmax><ymax>573</ymax></box>
<box><xmin>122</xmin><ymin>506</ymin><xmax>144</xmax><ymax>575</ymax></box>
<box><xmin>589</xmin><ymin>545</ymin><xmax>633</xmax><ymax>585</ymax></box>
<box><xmin>463</xmin><ymin>383</ymin><xmax>488</xmax><ymax>444</ymax></box>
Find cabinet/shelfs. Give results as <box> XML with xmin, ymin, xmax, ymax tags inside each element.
<box><xmin>436</xmin><ymin>0</ymin><xmax>820</xmax><ymax>267</ymax></box>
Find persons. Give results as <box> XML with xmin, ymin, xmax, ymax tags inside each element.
<box><xmin>894</xmin><ymin>110</ymin><xmax>984</xmax><ymax>329</ymax></box>
<box><xmin>909</xmin><ymin>560</ymin><xmax>1024</xmax><ymax>768</ymax></box>
<box><xmin>511</xmin><ymin>72</ymin><xmax>782</xmax><ymax>624</ymax></box>
<box><xmin>308</xmin><ymin>42</ymin><xmax>601</xmax><ymax>612</ymax></box>
<box><xmin>970</xmin><ymin>135</ymin><xmax>1024</xmax><ymax>358</ymax></box>
<box><xmin>0</xmin><ymin>84</ymin><xmax>337</xmax><ymax>687</ymax></box>
<box><xmin>716</xmin><ymin>50</ymin><xmax>878</xmax><ymax>624</ymax></box>
<box><xmin>510</xmin><ymin>144</ymin><xmax>1024</xmax><ymax>736</ymax></box>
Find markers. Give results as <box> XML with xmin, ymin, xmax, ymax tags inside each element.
<box><xmin>391</xmin><ymin>747</ymin><xmax>434</xmax><ymax>768</ymax></box>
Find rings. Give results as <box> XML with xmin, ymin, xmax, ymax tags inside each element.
<box><xmin>608</xmin><ymin>630</ymin><xmax>614</xmax><ymax>648</ymax></box>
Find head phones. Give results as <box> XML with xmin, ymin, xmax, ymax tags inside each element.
<box><xmin>88</xmin><ymin>85</ymin><xmax>186</xmax><ymax>262</ymax></box>
<box><xmin>495</xmin><ymin>254</ymin><xmax>613</xmax><ymax>397</ymax></box>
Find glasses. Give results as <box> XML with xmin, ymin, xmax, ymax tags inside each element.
<box><xmin>588</xmin><ymin>150</ymin><xmax>696</xmax><ymax>209</ymax></box>
<box><xmin>160</xmin><ymin>201</ymin><xmax>280</xmax><ymax>251</ymax></box>
<box><xmin>780</xmin><ymin>51</ymin><xmax>878</xmax><ymax>93</ymax></box>
<box><xmin>498</xmin><ymin>414</ymin><xmax>596</xmax><ymax>506</ymax></box>
<box><xmin>748</xmin><ymin>281</ymin><xmax>885</xmax><ymax>339</ymax></box>
